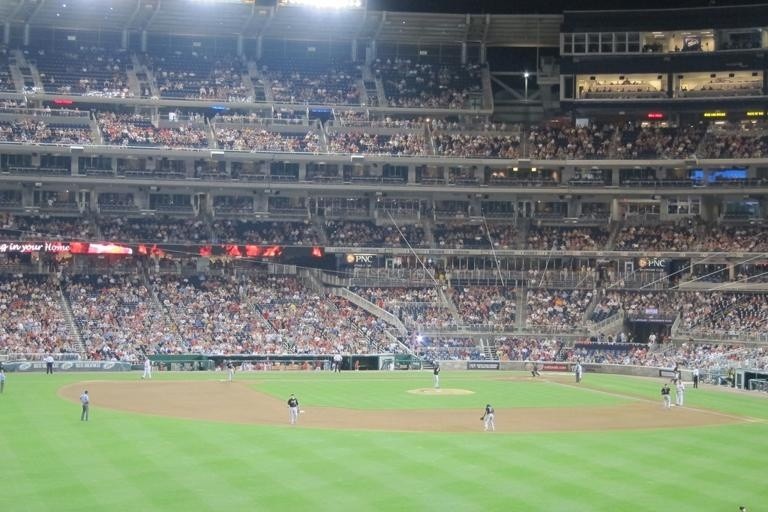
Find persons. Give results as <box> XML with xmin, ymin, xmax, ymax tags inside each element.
<box><xmin>80</xmin><ymin>390</ymin><xmax>91</xmax><ymax>419</ymax></box>
<box><xmin>673</xmin><ymin>366</ymin><xmax>681</xmax><ymax>384</ymax></box>
<box><xmin>574</xmin><ymin>358</ymin><xmax>583</xmax><ymax>383</ymax></box>
<box><xmin>2</xmin><ymin>42</ymin><xmax>483</xmax><ymax>109</ymax></box>
<box><xmin>4</xmin><ymin>196</ymin><xmax>765</xmax><ymax>288</ymax></box>
<box><xmin>286</xmin><ymin>394</ymin><xmax>300</xmax><ymax>425</ymax></box>
<box><xmin>481</xmin><ymin>404</ymin><xmax>495</xmax><ymax>431</ymax></box>
<box><xmin>433</xmin><ymin>361</ymin><xmax>440</xmax><ymax>388</ymax></box>
<box><xmin>142</xmin><ymin>357</ymin><xmax>151</xmax><ymax>378</ymax></box>
<box><xmin>412</xmin><ymin>274</ymin><xmax>766</xmax><ymax>370</ymax></box>
<box><xmin>661</xmin><ymin>383</ymin><xmax>671</xmax><ymax>408</ymax></box>
<box><xmin>0</xmin><ymin>370</ymin><xmax>5</xmax><ymax>392</ymax></box>
<box><xmin>676</xmin><ymin>381</ymin><xmax>683</xmax><ymax>405</ymax></box>
<box><xmin>46</xmin><ymin>354</ymin><xmax>54</xmax><ymax>374</ymax></box>
<box><xmin>2</xmin><ymin>271</ymin><xmax>411</xmax><ymax>371</ymax></box>
<box><xmin>334</xmin><ymin>351</ymin><xmax>343</xmax><ymax>373</ymax></box>
<box><xmin>691</xmin><ymin>365</ymin><xmax>701</xmax><ymax>389</ymax></box>
<box><xmin>531</xmin><ymin>362</ymin><xmax>540</xmax><ymax>376</ymax></box>
<box><xmin>3</xmin><ymin>101</ymin><xmax>765</xmax><ymax>185</ymax></box>
<box><xmin>227</xmin><ymin>362</ymin><xmax>234</xmax><ymax>377</ymax></box>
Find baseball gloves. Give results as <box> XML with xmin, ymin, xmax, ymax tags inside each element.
<box><xmin>480</xmin><ymin>417</ymin><xmax>484</xmax><ymax>420</ymax></box>
